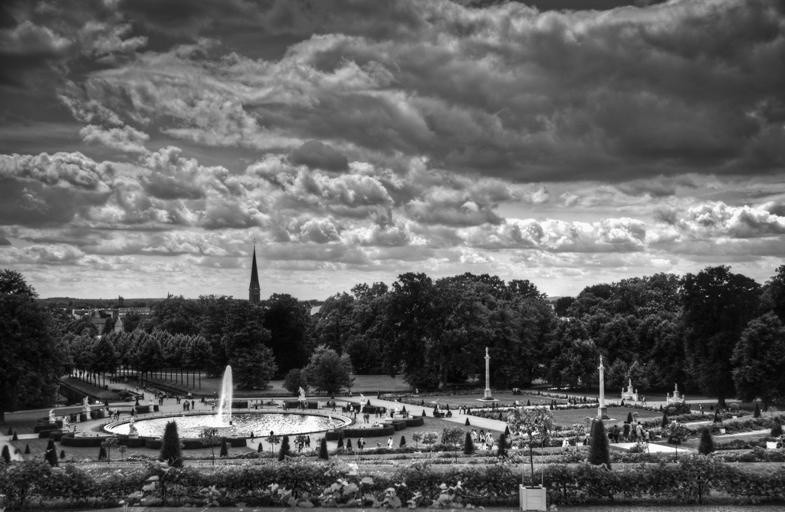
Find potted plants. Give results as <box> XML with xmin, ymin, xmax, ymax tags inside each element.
<box><xmin>507</xmin><ymin>408</ymin><xmax>553</xmax><ymax>512</ymax></box>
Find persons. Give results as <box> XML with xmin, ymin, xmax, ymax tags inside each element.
<box><xmin>62</xmin><ymin>375</ymin><xmax>785</xmax><ymax>455</ymax></box>
